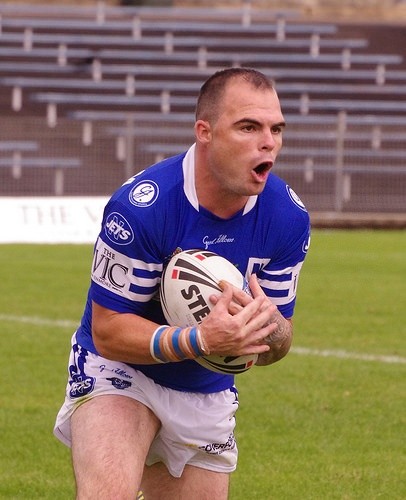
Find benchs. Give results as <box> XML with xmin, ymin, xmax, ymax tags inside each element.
<box><xmin>0</xmin><ymin>4</ymin><xmax>406</xmax><ymax>201</ymax></box>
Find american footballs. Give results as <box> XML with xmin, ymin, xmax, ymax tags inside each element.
<box><xmin>159</xmin><ymin>249</ymin><xmax>258</xmax><ymax>375</ymax></box>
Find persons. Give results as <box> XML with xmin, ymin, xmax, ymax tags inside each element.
<box><xmin>50</xmin><ymin>67</ymin><xmax>311</xmax><ymax>500</ymax></box>
